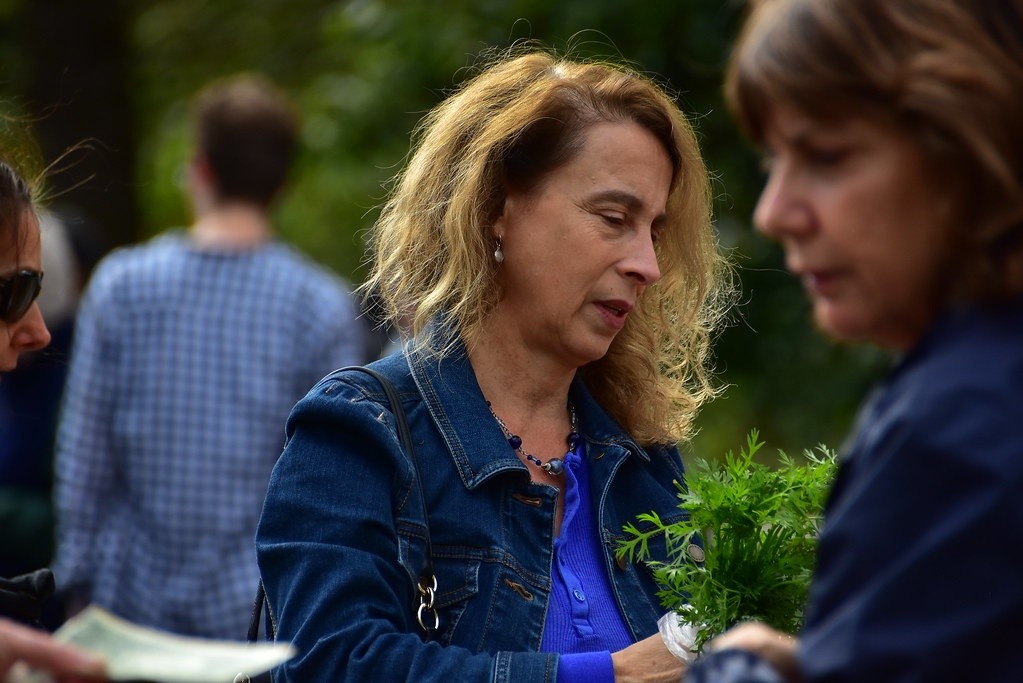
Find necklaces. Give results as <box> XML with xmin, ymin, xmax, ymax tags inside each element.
<box><xmin>484</xmin><ymin>396</ymin><xmax>578</xmax><ymax>475</ymax></box>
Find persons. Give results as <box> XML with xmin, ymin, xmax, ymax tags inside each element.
<box><xmin>255</xmin><ymin>49</ymin><xmax>730</xmax><ymax>683</ymax></box>
<box><xmin>687</xmin><ymin>1</ymin><xmax>1023</xmax><ymax>683</ymax></box>
<box><xmin>1</xmin><ymin>75</ymin><xmax>367</xmax><ymax>682</ymax></box>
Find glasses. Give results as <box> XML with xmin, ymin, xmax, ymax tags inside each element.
<box><xmin>0</xmin><ymin>269</ymin><xmax>44</xmax><ymax>324</ymax></box>
